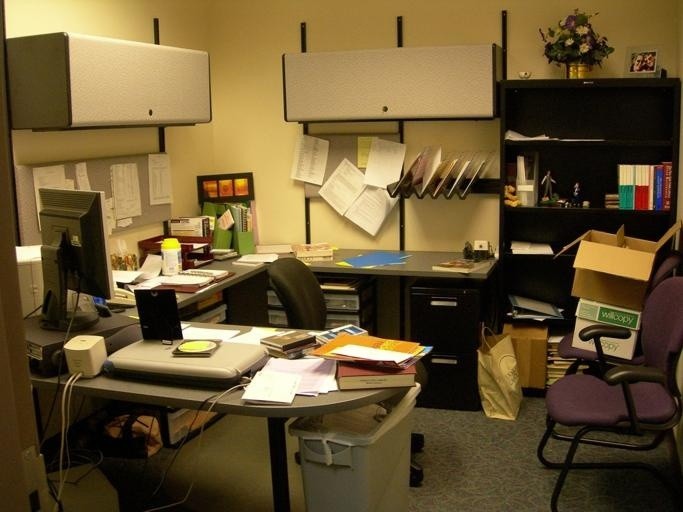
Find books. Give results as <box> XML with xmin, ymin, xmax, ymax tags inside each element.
<box><xmin>432</xmin><ymin>258</ymin><xmax>491</xmax><ymax>274</ymax></box>
<box><xmin>168</xmin><ymin>205</ymin><xmax>252</xmax><ymax>254</ymax></box>
<box><xmin>242</xmin><ymin>324</ymin><xmax>433</xmax><ymax>405</ymax></box>
<box><xmin>618</xmin><ymin>162</ymin><xmax>672</xmax><ymax>210</ymax></box>
<box><xmin>546</xmin><ymin>335</ymin><xmax>589</xmax><ymax>390</ymax></box>
<box><xmin>150</xmin><ymin>269</ymin><xmax>236</xmax><ymax>293</ymax></box>
<box><xmin>292</xmin><ymin>243</ymin><xmax>334</xmax><ymax>261</ymax></box>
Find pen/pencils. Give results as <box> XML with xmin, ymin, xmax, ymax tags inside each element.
<box><xmin>110</xmin><ymin>253</ymin><xmax>139</xmax><ymax>270</ymax></box>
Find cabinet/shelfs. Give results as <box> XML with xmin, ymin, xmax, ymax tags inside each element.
<box><xmin>282</xmin><ymin>43</ymin><xmax>504</xmax><ymax>123</ymax></box>
<box><xmin>498</xmin><ymin>78</ymin><xmax>681</xmax><ymax>311</ymax></box>
<box><xmin>5</xmin><ymin>32</ymin><xmax>212</xmax><ymax>132</ymax></box>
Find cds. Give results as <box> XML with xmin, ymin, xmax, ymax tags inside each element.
<box><xmin>178</xmin><ymin>341</ymin><xmax>217</xmax><ymax>352</ymax></box>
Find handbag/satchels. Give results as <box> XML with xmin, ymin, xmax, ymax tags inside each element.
<box><xmin>477</xmin><ymin>326</ymin><xmax>524</xmax><ymax>420</ymax></box>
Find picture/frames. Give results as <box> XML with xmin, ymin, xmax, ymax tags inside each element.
<box><xmin>630</xmin><ymin>50</ymin><xmax>658</xmax><ymax>73</ymax></box>
<box><xmin>197</xmin><ymin>172</ymin><xmax>254</xmax><ymax>205</ymax></box>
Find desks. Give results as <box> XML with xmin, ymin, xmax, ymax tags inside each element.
<box><xmin>33</xmin><ymin>249</ymin><xmax>268</xmax><ymax>445</ymax></box>
<box><xmin>29</xmin><ymin>318</ymin><xmax>416</xmax><ymax>512</ymax></box>
<box><xmin>273</xmin><ymin>245</ymin><xmax>499</xmax><ymax>384</ymax></box>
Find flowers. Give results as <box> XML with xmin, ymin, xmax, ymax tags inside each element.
<box><xmin>538</xmin><ymin>7</ymin><xmax>616</xmax><ymax>59</ymax></box>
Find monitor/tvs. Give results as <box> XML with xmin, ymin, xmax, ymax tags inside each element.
<box><xmin>38</xmin><ymin>187</ymin><xmax>114</xmax><ymax>331</ymax></box>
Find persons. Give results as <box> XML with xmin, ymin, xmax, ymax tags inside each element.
<box><xmin>572</xmin><ymin>182</ymin><xmax>581</xmax><ymax>207</ymax></box>
<box><xmin>630</xmin><ymin>52</ymin><xmax>655</xmax><ymax>72</ymax></box>
<box><xmin>541</xmin><ymin>169</ymin><xmax>556</xmax><ymax>200</ymax></box>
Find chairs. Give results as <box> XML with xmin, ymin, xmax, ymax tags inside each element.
<box><xmin>538</xmin><ymin>276</ymin><xmax>683</xmax><ymax>511</ymax></box>
<box><xmin>268</xmin><ymin>257</ymin><xmax>328</xmax><ymax>330</ymax></box>
<box><xmin>542</xmin><ymin>251</ymin><xmax>683</xmax><ymax>451</ymax></box>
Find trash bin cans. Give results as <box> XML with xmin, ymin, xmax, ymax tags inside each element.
<box><xmin>288</xmin><ymin>382</ymin><xmax>421</xmax><ymax>512</ymax></box>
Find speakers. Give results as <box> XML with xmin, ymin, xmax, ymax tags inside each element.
<box><xmin>63</xmin><ymin>335</ymin><xmax>107</xmax><ymax>378</ymax></box>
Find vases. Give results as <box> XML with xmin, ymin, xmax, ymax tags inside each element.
<box><xmin>566</xmin><ymin>62</ymin><xmax>589</xmax><ymax>79</ymax></box>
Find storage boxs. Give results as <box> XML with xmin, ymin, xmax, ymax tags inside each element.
<box><xmin>502</xmin><ymin>322</ymin><xmax>545</xmax><ymax>389</ymax></box>
<box><xmin>571</xmin><ymin>297</ymin><xmax>642</xmax><ymax>360</ymax></box>
<box><xmin>552</xmin><ymin>220</ymin><xmax>683</xmax><ymax>312</ymax></box>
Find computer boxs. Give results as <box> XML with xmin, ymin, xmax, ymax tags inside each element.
<box><xmin>22</xmin><ymin>306</ymin><xmax>143</xmax><ymax>378</ymax></box>
